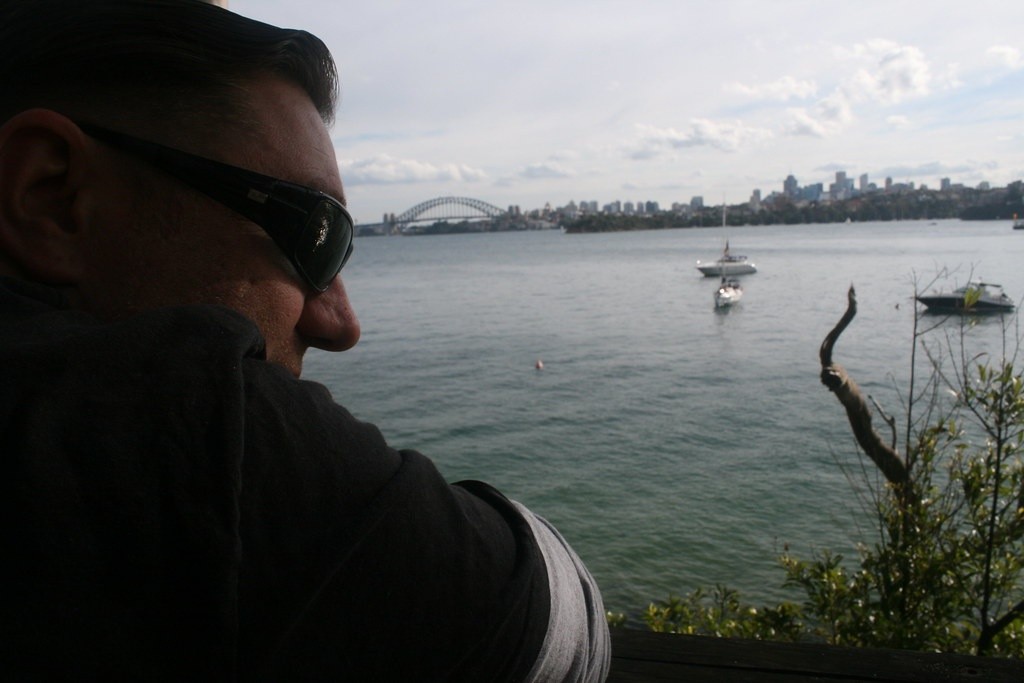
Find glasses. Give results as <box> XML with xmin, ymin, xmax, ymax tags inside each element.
<box><xmin>68</xmin><ymin>118</ymin><xmax>356</xmax><ymax>293</ymax></box>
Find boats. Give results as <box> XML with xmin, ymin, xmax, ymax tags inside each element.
<box><xmin>1012</xmin><ymin>219</ymin><xmax>1024</xmax><ymax>230</ymax></box>
<box><xmin>917</xmin><ymin>282</ymin><xmax>1015</xmax><ymax>313</ymax></box>
<box><xmin>713</xmin><ymin>278</ymin><xmax>743</xmax><ymax>308</ymax></box>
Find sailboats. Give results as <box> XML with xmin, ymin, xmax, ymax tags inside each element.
<box><xmin>697</xmin><ymin>194</ymin><xmax>758</xmax><ymax>277</ymax></box>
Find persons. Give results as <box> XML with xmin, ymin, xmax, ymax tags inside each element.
<box><xmin>0</xmin><ymin>0</ymin><xmax>612</xmax><ymax>683</ymax></box>
